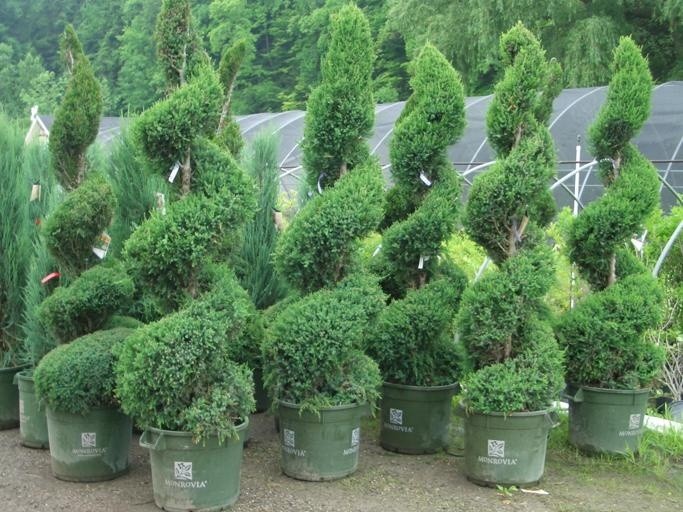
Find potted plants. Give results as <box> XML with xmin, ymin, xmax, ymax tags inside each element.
<box><xmin>373</xmin><ymin>41</ymin><xmax>467</xmax><ymax>455</ymax></box>
<box><xmin>562</xmin><ymin>40</ymin><xmax>667</xmax><ymax>458</ymax></box>
<box><xmin>459</xmin><ymin>34</ymin><xmax>568</xmax><ymax>488</ymax></box>
<box><xmin>112</xmin><ymin>62</ymin><xmax>260</xmax><ymax>512</ymax></box>
<box><xmin>1</xmin><ymin>61</ymin><xmax>40</xmax><ymax>428</ymax></box>
<box><xmin>14</xmin><ymin>139</ymin><xmax>88</xmax><ymax>449</ymax></box>
<box><xmin>261</xmin><ymin>35</ymin><xmax>389</xmax><ymax>481</ymax></box>
<box><xmin>33</xmin><ymin>67</ymin><xmax>113</xmax><ymax>482</ymax></box>
